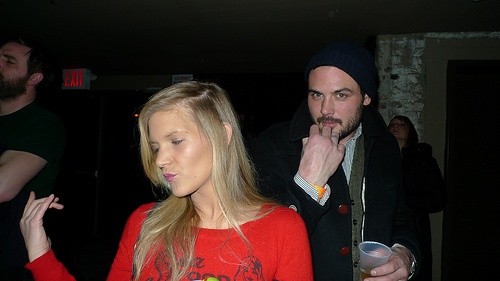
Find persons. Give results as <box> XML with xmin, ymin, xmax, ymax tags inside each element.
<box><xmin>1</xmin><ymin>32</ymin><xmax>63</xmax><ymax>281</ymax></box>
<box><xmin>19</xmin><ymin>79</ymin><xmax>314</xmax><ymax>280</ymax></box>
<box><xmin>246</xmin><ymin>41</ymin><xmax>420</xmax><ymax>281</ymax></box>
<box><xmin>388</xmin><ymin>115</ymin><xmax>448</xmax><ymax>280</ymax></box>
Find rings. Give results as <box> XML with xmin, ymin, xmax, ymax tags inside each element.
<box><xmin>332</xmin><ymin>135</ymin><xmax>339</xmax><ymax>140</ymax></box>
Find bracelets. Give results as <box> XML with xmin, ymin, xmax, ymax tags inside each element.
<box><xmin>391</xmin><ymin>246</ymin><xmax>416</xmax><ymax>276</ymax></box>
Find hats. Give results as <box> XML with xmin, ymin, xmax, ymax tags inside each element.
<box><xmin>305</xmin><ymin>41</ymin><xmax>380</xmax><ymax>101</ymax></box>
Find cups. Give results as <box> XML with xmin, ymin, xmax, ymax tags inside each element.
<box><xmin>357</xmin><ymin>241</ymin><xmax>393</xmax><ymax>281</ymax></box>
